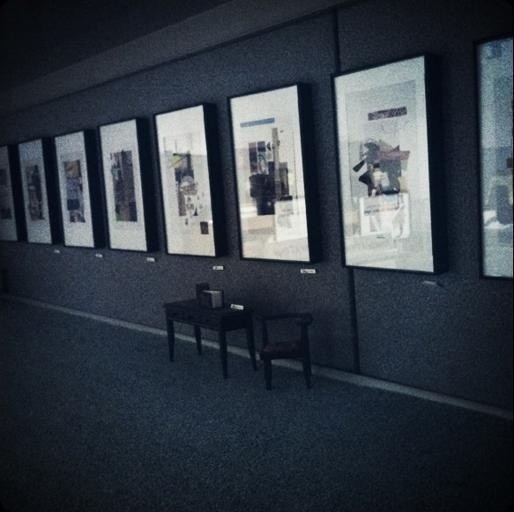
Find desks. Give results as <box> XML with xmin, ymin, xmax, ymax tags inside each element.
<box><xmin>163</xmin><ymin>298</ymin><xmax>257</xmax><ymax>383</ymax></box>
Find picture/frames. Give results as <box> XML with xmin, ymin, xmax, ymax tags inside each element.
<box><xmin>226</xmin><ymin>82</ymin><xmax>324</xmax><ymax>264</ymax></box>
<box><xmin>329</xmin><ymin>51</ymin><xmax>450</xmax><ymax>276</ymax></box>
<box><xmin>97</xmin><ymin>117</ymin><xmax>160</xmax><ymax>254</ymax></box>
<box><xmin>17</xmin><ymin>136</ymin><xmax>63</xmax><ymax>245</ymax></box>
<box><xmin>152</xmin><ymin>102</ymin><xmax>225</xmax><ymax>259</ymax></box>
<box><xmin>473</xmin><ymin>28</ymin><xmax>514</xmax><ymax>281</ymax></box>
<box><xmin>52</xmin><ymin>127</ymin><xmax>105</xmax><ymax>250</ymax></box>
<box><xmin>0</xmin><ymin>144</ymin><xmax>25</xmax><ymax>242</ymax></box>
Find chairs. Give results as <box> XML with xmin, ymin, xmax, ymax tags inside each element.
<box><xmin>248</xmin><ymin>312</ymin><xmax>313</xmax><ymax>391</ymax></box>
